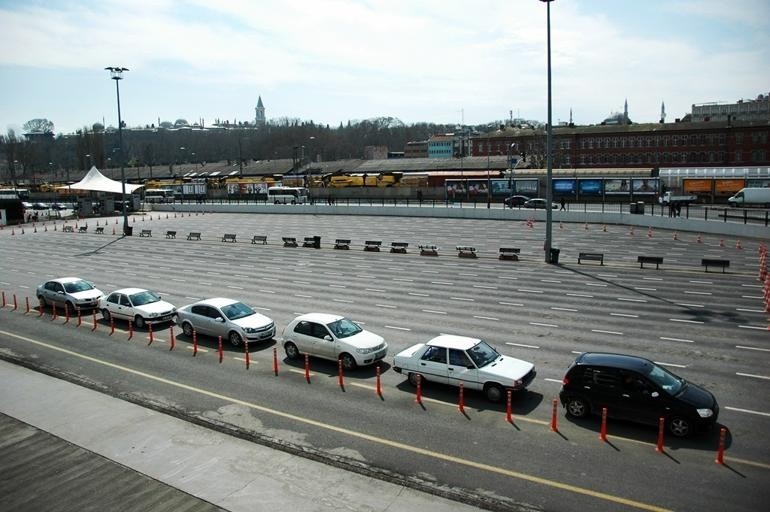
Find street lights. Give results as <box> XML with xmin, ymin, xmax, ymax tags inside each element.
<box><xmin>102</xmin><ymin>65</ymin><xmax>132</xmax><ymax>235</ymax></box>
<box><xmin>538</xmin><ymin>0</ymin><xmax>555</xmax><ymax>264</ymax></box>
<box><xmin>509</xmin><ymin>140</ymin><xmax>525</xmax><ymax>200</ymax></box>
<box><xmin>442</xmin><ymin>131</ymin><xmax>464</xmax><ymax>179</ymax></box>
<box><xmin>8</xmin><ymin>146</ymin><xmax>201</xmax><ymax>179</ymax></box>
<box><xmin>484</xmin><ymin>122</ymin><xmax>492</xmax><ymax>209</ymax></box>
<box><xmin>301</xmin><ymin>136</ymin><xmax>316</xmax><ymax>163</ymax></box>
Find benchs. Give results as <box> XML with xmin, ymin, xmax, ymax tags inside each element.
<box><xmin>637</xmin><ymin>256</ymin><xmax>663</xmax><ymax>270</ymax></box>
<box><xmin>63</xmin><ymin>225</ymin><xmax>267</xmax><ymax>245</ymax></box>
<box><xmin>418</xmin><ymin>244</ymin><xmax>440</xmax><ymax>256</ymax></box>
<box><xmin>282</xmin><ymin>238</ymin><xmax>298</xmax><ymax>247</ymax></box>
<box><xmin>390</xmin><ymin>243</ymin><xmax>408</xmax><ymax>253</ymax></box>
<box><xmin>456</xmin><ymin>246</ymin><xmax>476</xmax><ymax>257</ymax></box>
<box><xmin>364</xmin><ymin>241</ymin><xmax>382</xmax><ymax>252</ymax></box>
<box><xmin>578</xmin><ymin>253</ymin><xmax>603</xmax><ymax>266</ymax></box>
<box><xmin>701</xmin><ymin>259</ymin><xmax>729</xmax><ymax>273</ymax></box>
<box><xmin>499</xmin><ymin>248</ymin><xmax>520</xmax><ymax>261</ymax></box>
<box><xmin>332</xmin><ymin>239</ymin><xmax>351</xmax><ymax>250</ymax></box>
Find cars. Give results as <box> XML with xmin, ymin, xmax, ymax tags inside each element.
<box><xmin>174</xmin><ymin>296</ymin><xmax>277</xmax><ymax>349</ymax></box>
<box><xmin>92</xmin><ymin>286</ymin><xmax>178</xmax><ymax>331</ymax></box>
<box><xmin>35</xmin><ymin>274</ymin><xmax>105</xmax><ymax>315</ymax></box>
<box><xmin>0</xmin><ymin>187</ymin><xmax>67</xmax><ymax>211</ymax></box>
<box><xmin>504</xmin><ymin>195</ymin><xmax>528</xmax><ymax>208</ymax></box>
<box><xmin>523</xmin><ymin>197</ymin><xmax>559</xmax><ymax>209</ymax></box>
<box><xmin>391</xmin><ymin>330</ymin><xmax>539</xmax><ymax>403</ymax></box>
<box><xmin>279</xmin><ymin>310</ymin><xmax>391</xmax><ymax>371</ymax></box>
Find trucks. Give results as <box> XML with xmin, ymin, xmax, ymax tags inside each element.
<box><xmin>727</xmin><ymin>186</ymin><xmax>770</xmax><ymax>208</ymax></box>
<box><xmin>658</xmin><ymin>192</ymin><xmax>699</xmax><ymax>207</ymax></box>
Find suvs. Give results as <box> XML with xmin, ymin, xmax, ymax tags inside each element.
<box><xmin>554</xmin><ymin>350</ymin><xmax>722</xmax><ymax>439</ymax></box>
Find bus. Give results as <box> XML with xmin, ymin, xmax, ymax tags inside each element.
<box><xmin>111</xmin><ymin>200</ymin><xmax>131</xmax><ymax>215</ymax></box>
<box><xmin>266</xmin><ymin>185</ymin><xmax>311</xmax><ymax>205</ymax></box>
<box><xmin>143</xmin><ymin>187</ymin><xmax>175</xmax><ymax>204</ymax></box>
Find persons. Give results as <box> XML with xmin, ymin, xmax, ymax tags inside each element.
<box><xmin>619</xmin><ymin>180</ymin><xmax>628</xmax><ymax>191</ymax></box>
<box><xmin>639</xmin><ymin>181</ymin><xmax>655</xmax><ymax>191</ymax></box>
<box><xmin>671</xmin><ymin>200</ymin><xmax>681</xmax><ymax>218</ymax></box>
<box><xmin>559</xmin><ymin>197</ymin><xmax>566</xmax><ymax>211</ymax></box>
